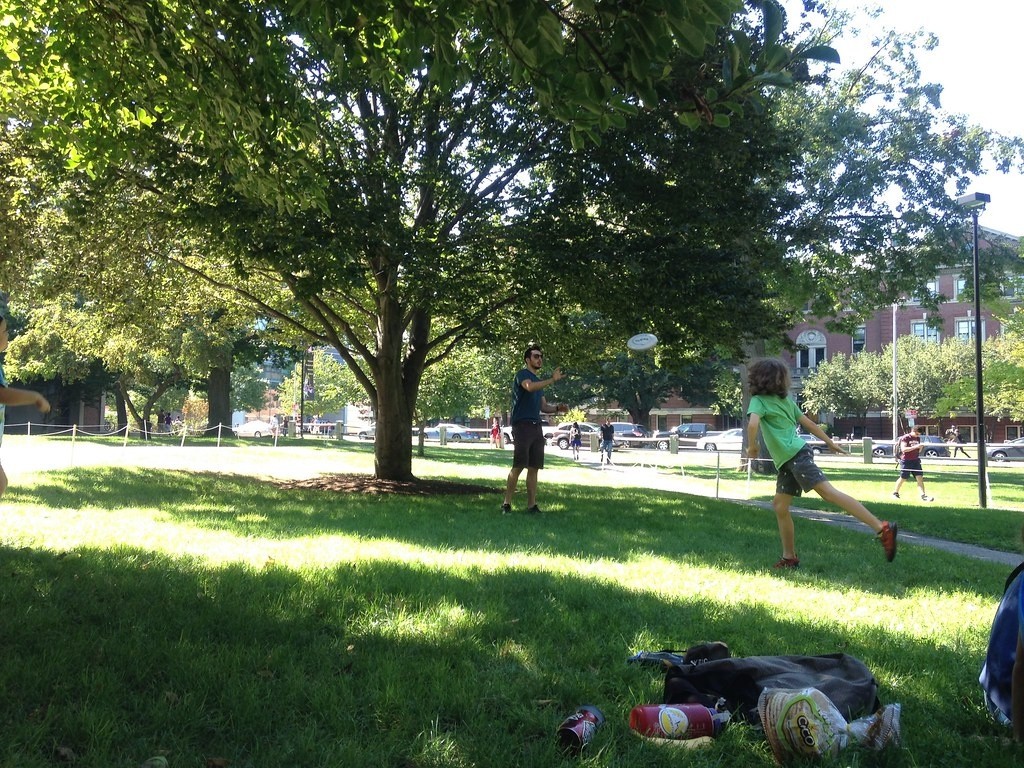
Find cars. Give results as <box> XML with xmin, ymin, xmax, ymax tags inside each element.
<box><xmin>986</xmin><ymin>435</ymin><xmax>1024</xmax><ymax>463</ymax></box>
<box><xmin>873</xmin><ymin>434</ymin><xmax>951</xmax><ymax>459</ymax></box>
<box><xmin>797</xmin><ymin>434</ymin><xmax>834</xmax><ymax>456</ymax></box>
<box><xmin>503</xmin><ymin>419</ymin><xmax>555</xmax><ymax>445</ymax></box>
<box><xmin>417</xmin><ymin>422</ymin><xmax>481</xmax><ymax>443</ymax></box>
<box><xmin>357</xmin><ymin>423</ymin><xmax>377</xmax><ymax>440</ymax></box>
<box><xmin>234</xmin><ymin>420</ymin><xmax>276</xmax><ymax>438</ymax></box>
<box><xmin>697</xmin><ymin>427</ymin><xmax>744</xmax><ymax>452</ymax></box>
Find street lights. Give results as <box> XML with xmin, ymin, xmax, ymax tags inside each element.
<box><xmin>955</xmin><ymin>192</ymin><xmax>993</xmax><ymax>509</ymax></box>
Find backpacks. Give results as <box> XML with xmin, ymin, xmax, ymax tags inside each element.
<box><xmin>893</xmin><ymin>436</ymin><xmax>908</xmax><ymax>458</ymax></box>
<box><xmin>572</xmin><ymin>427</ymin><xmax>580</xmax><ymax>438</ymax></box>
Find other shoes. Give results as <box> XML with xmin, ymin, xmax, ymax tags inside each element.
<box><xmin>893</xmin><ymin>492</ymin><xmax>900</xmax><ymax>499</ymax></box>
<box><xmin>529</xmin><ymin>505</ymin><xmax>541</xmax><ymax>513</ymax></box>
<box><xmin>501</xmin><ymin>504</ymin><xmax>512</xmax><ymax>514</ymax></box>
<box><xmin>921</xmin><ymin>494</ymin><xmax>934</xmax><ymax>501</ymax></box>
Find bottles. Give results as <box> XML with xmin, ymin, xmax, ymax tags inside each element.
<box><xmin>558</xmin><ymin>705</ymin><xmax>606</xmax><ymax>755</ymax></box>
<box><xmin>629</xmin><ymin>705</ymin><xmax>721</xmax><ymax>740</ymax></box>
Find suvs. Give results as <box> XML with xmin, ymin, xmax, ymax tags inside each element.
<box><xmin>597</xmin><ymin>421</ymin><xmax>650</xmax><ymax>448</ymax></box>
<box><xmin>551</xmin><ymin>421</ymin><xmax>604</xmax><ymax>450</ymax></box>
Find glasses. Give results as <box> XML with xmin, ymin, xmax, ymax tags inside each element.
<box><xmin>531</xmin><ymin>354</ymin><xmax>544</xmax><ymax>359</ymax></box>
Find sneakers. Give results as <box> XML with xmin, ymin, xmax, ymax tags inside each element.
<box><xmin>875</xmin><ymin>521</ymin><xmax>897</xmax><ymax>562</ymax></box>
<box><xmin>774</xmin><ymin>555</ymin><xmax>799</xmax><ymax>568</ymax></box>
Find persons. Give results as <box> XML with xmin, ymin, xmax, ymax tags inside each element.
<box><xmin>599</xmin><ymin>416</ymin><xmax>614</xmax><ymax>464</ymax></box>
<box><xmin>501</xmin><ymin>346</ymin><xmax>567</xmax><ymax>514</ymax></box>
<box><xmin>0</xmin><ymin>315</ymin><xmax>50</xmax><ymax>495</ymax></box>
<box><xmin>892</xmin><ymin>425</ymin><xmax>934</xmax><ymax>501</ymax></box>
<box><xmin>570</xmin><ymin>421</ymin><xmax>582</xmax><ymax>460</ymax></box>
<box><xmin>954</xmin><ymin>429</ymin><xmax>971</xmax><ymax>458</ymax></box>
<box><xmin>491</xmin><ymin>424</ymin><xmax>500</xmax><ymax>448</ymax></box>
<box><xmin>796</xmin><ymin>424</ymin><xmax>803</xmax><ymax>435</ymax></box>
<box><xmin>158</xmin><ymin>409</ymin><xmax>172</xmax><ymax>432</ymax></box>
<box><xmin>745</xmin><ymin>356</ymin><xmax>898</xmax><ymax>567</ymax></box>
<box><xmin>271</xmin><ymin>414</ymin><xmax>278</xmax><ymax>432</ymax></box>
<box><xmin>176</xmin><ymin>415</ymin><xmax>181</xmax><ymax>422</ymax></box>
<box><xmin>283</xmin><ymin>416</ymin><xmax>288</xmax><ymax>436</ymax></box>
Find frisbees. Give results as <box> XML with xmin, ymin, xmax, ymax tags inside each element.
<box><xmin>626</xmin><ymin>333</ymin><xmax>658</xmax><ymax>352</ymax></box>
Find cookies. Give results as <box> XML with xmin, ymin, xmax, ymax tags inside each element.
<box><xmin>765</xmin><ymin>687</ymin><xmax>842</xmax><ymax>767</ymax></box>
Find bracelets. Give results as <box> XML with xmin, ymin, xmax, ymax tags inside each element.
<box><xmin>557</xmin><ymin>405</ymin><xmax>558</xmax><ymax>410</ymax></box>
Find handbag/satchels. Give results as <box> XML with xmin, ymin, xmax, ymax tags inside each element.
<box><xmin>661</xmin><ymin>643</ymin><xmax>881</xmax><ymax>734</ymax></box>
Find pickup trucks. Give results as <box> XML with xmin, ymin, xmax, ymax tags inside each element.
<box><xmin>654</xmin><ymin>422</ymin><xmax>725</xmax><ymax>451</ymax></box>
